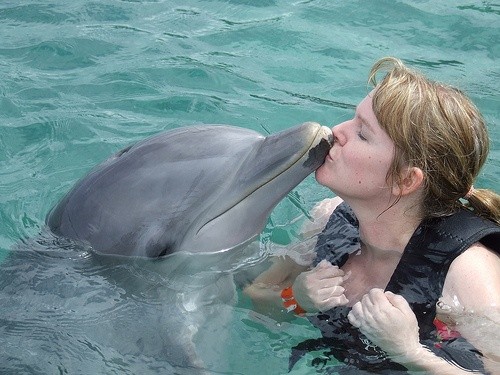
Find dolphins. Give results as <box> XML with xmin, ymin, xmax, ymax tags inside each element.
<box><xmin>0</xmin><ymin>122</ymin><xmax>333</xmax><ymax>375</ymax></box>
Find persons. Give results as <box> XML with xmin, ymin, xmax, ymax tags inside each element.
<box><xmin>244</xmin><ymin>53</ymin><xmax>500</xmax><ymax>374</ymax></box>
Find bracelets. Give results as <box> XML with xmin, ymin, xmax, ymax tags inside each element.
<box><xmin>280</xmin><ymin>286</ymin><xmax>306</xmax><ymax>317</ymax></box>
